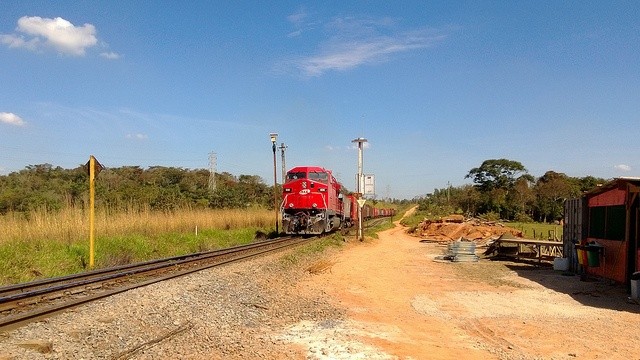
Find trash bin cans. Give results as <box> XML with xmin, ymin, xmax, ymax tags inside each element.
<box><xmin>631</xmin><ymin>271</ymin><xmax>640</xmax><ymax>305</ymax></box>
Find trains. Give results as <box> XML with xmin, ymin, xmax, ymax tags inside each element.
<box><xmin>281</xmin><ymin>167</ymin><xmax>396</xmax><ymax>238</ymax></box>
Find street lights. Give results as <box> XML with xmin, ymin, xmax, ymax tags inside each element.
<box><xmin>352</xmin><ymin>138</ymin><xmax>366</xmax><ymax>241</ymax></box>
<box><xmin>270</xmin><ymin>133</ymin><xmax>278</xmax><ymax>232</ymax></box>
